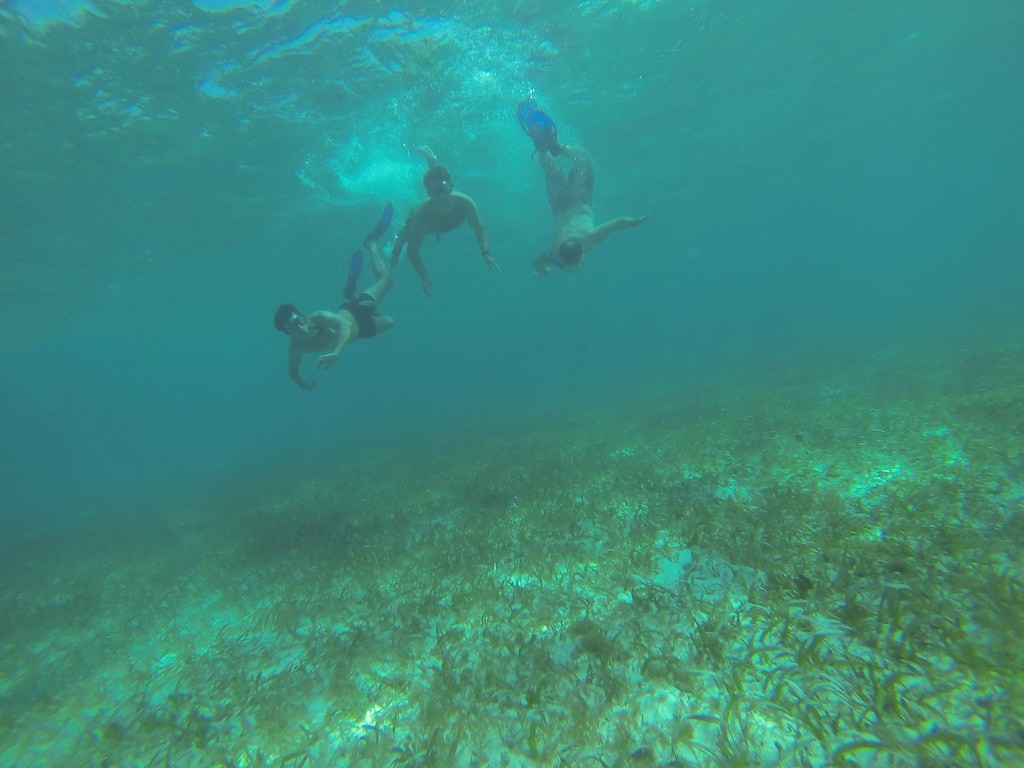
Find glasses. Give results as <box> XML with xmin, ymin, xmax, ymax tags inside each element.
<box><xmin>427</xmin><ymin>179</ymin><xmax>447</xmax><ymax>194</ymax></box>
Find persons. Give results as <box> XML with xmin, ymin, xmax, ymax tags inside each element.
<box><xmin>272</xmin><ymin>241</ymin><xmax>396</xmax><ymax>389</ymax></box>
<box><xmin>518</xmin><ymin>101</ymin><xmax>648</xmax><ymax>278</ymax></box>
<box><xmin>385</xmin><ymin>144</ymin><xmax>502</xmax><ymax>297</ymax></box>
